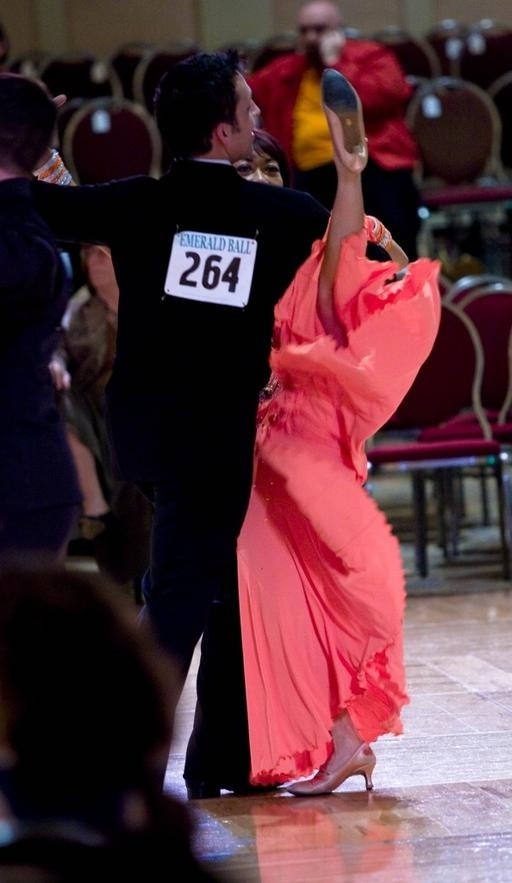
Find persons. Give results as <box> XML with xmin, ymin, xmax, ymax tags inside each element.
<box><xmin>1</xmin><ymin>1</ymin><xmax>440</xmax><ymax>791</ymax></box>
<box><xmin>1</xmin><ymin>553</ymin><xmax>239</xmax><ymax>882</ymax></box>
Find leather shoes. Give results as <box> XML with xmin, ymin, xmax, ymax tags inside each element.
<box><xmin>184</xmin><ymin>772</ymin><xmax>282</xmax><ymax>798</ymax></box>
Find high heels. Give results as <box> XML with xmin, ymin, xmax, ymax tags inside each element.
<box><xmin>284</xmin><ymin>742</ymin><xmax>377</xmax><ymax>796</ymax></box>
<box><xmin>318</xmin><ymin>66</ymin><xmax>371</xmax><ymax>174</ymax></box>
